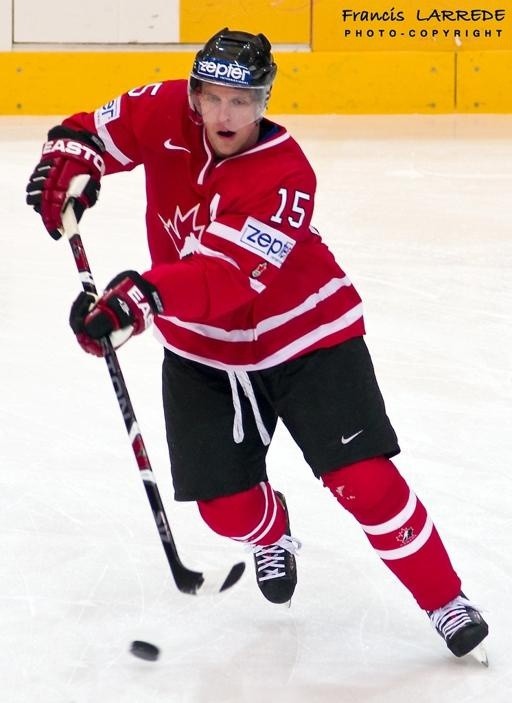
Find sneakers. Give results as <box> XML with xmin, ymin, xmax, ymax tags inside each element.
<box><xmin>427</xmin><ymin>590</ymin><xmax>489</xmax><ymax>658</ymax></box>
<box><xmin>253</xmin><ymin>490</ymin><xmax>297</xmax><ymax>604</ymax></box>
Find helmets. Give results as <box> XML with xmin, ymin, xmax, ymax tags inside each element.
<box><xmin>189</xmin><ymin>27</ymin><xmax>278</xmax><ymax>89</ymax></box>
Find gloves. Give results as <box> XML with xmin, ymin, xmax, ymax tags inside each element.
<box><xmin>69</xmin><ymin>270</ymin><xmax>164</xmax><ymax>357</ymax></box>
<box><xmin>26</xmin><ymin>125</ymin><xmax>106</xmax><ymax>241</ymax></box>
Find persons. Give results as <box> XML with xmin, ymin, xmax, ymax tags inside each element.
<box><xmin>24</xmin><ymin>28</ymin><xmax>488</xmax><ymax>657</ymax></box>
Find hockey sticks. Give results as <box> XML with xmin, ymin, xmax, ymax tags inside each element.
<box><xmin>60</xmin><ymin>203</ymin><xmax>245</xmax><ymax>595</ymax></box>
